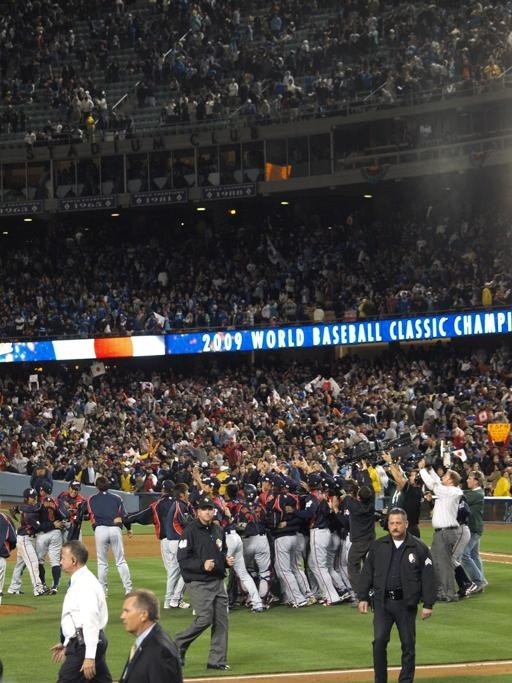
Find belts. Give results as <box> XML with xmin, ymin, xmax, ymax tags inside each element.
<box><xmin>435</xmin><ymin>526</ymin><xmax>458</xmax><ymax>531</ymax></box>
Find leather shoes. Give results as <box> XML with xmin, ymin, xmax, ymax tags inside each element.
<box><xmin>207</xmin><ymin>663</ymin><xmax>230</xmax><ymax>670</ymax></box>
<box><xmin>178</xmin><ymin>648</ymin><xmax>185</xmax><ymax>666</ymax></box>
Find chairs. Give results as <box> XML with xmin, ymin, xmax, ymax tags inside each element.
<box><xmin>0</xmin><ymin>7</ymin><xmax>332</xmax><ymax>148</ymax></box>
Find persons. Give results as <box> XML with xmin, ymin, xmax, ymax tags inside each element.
<box><xmin>50</xmin><ymin>541</ymin><xmax>112</xmax><ymax>683</ymax></box>
<box><xmin>118</xmin><ymin>588</ymin><xmax>183</xmax><ymax>683</ymax></box>
<box><xmin>0</xmin><ymin>660</ymin><xmax>3</xmax><ymax>683</ymax></box>
<box><xmin>1</xmin><ymin>335</ymin><xmax>512</xmax><ymax>494</ymax></box>
<box><xmin>357</xmin><ymin>508</ymin><xmax>436</xmax><ymax>683</ymax></box>
<box><xmin>1</xmin><ymin>135</ymin><xmax>512</xmax><ymax>341</ymax></box>
<box><xmin>172</xmin><ymin>498</ymin><xmax>231</xmax><ymax>672</ymax></box>
<box><xmin>1</xmin><ymin>455</ymin><xmax>489</xmax><ymax>611</ymax></box>
<box><xmin>1</xmin><ymin>1</ymin><xmax>512</xmax><ymax>145</ymax></box>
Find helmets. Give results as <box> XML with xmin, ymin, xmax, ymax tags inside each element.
<box><xmin>244</xmin><ymin>483</ymin><xmax>257</xmax><ymax>495</ymax></box>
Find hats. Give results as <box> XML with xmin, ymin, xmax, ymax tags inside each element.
<box><xmin>23</xmin><ymin>480</ymin><xmax>81</xmax><ymax>497</ymax></box>
<box><xmin>161</xmin><ymin>480</ymin><xmax>176</xmax><ymax>491</ymax></box>
<box><xmin>470</xmin><ymin>471</ymin><xmax>484</xmax><ymax>489</ymax></box>
<box><xmin>199</xmin><ymin>496</ymin><xmax>214</xmax><ymax>509</ymax></box>
<box><xmin>201</xmin><ymin>477</ymin><xmax>220</xmax><ymax>489</ymax></box>
<box><xmin>280</xmin><ymin>474</ymin><xmax>344</xmax><ymax>488</ymax></box>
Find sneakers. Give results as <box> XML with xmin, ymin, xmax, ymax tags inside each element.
<box><xmin>8</xmin><ymin>584</ymin><xmax>58</xmax><ymax>595</ymax></box>
<box><xmin>227</xmin><ymin>589</ymin><xmax>358</xmax><ymax>612</ymax></box>
<box><xmin>163</xmin><ymin>599</ymin><xmax>191</xmax><ymax>609</ymax></box>
<box><xmin>436</xmin><ymin>582</ymin><xmax>488</xmax><ymax>601</ymax></box>
